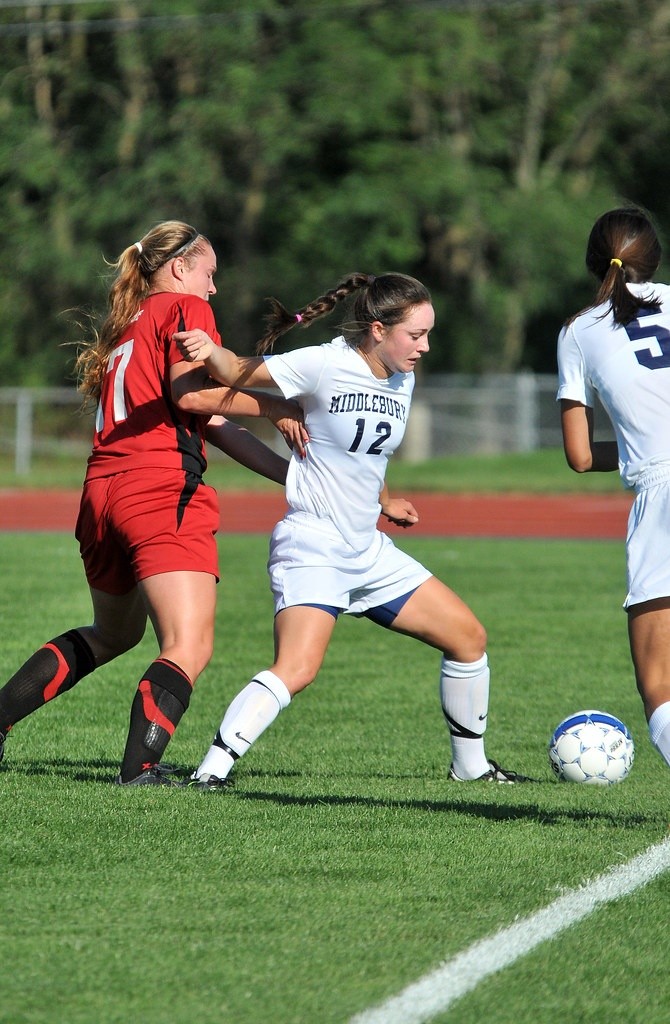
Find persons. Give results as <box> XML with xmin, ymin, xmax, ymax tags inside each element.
<box><xmin>0</xmin><ymin>218</ymin><xmax>417</xmax><ymax>783</ymax></box>
<box><xmin>557</xmin><ymin>203</ymin><xmax>670</xmax><ymax>778</ymax></box>
<box><xmin>165</xmin><ymin>272</ymin><xmax>527</xmax><ymax>792</ymax></box>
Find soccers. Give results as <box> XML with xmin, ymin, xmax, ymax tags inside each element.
<box><xmin>549</xmin><ymin>709</ymin><xmax>636</xmax><ymax>785</ymax></box>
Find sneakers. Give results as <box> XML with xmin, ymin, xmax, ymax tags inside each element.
<box><xmin>0</xmin><ymin>730</ymin><xmax>232</xmax><ymax>792</ymax></box>
<box><xmin>447</xmin><ymin>762</ymin><xmax>529</xmax><ymax>783</ymax></box>
